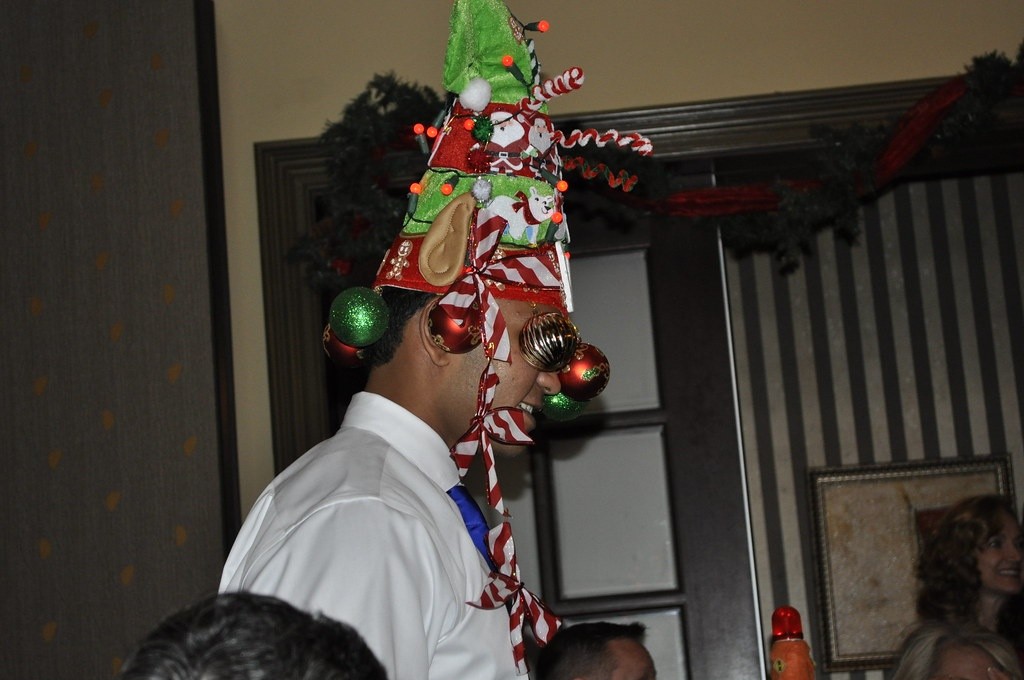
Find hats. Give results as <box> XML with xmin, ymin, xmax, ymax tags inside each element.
<box><xmin>375</xmin><ymin>0</ymin><xmax>574</xmax><ymax>309</ymax></box>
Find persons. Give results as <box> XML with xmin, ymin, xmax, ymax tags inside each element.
<box><xmin>910</xmin><ymin>494</ymin><xmax>1024</xmax><ymax>633</ymax></box>
<box><xmin>534</xmin><ymin>620</ymin><xmax>658</xmax><ymax>680</ymax></box>
<box><xmin>890</xmin><ymin>617</ymin><xmax>1023</xmax><ymax>680</ymax></box>
<box><xmin>215</xmin><ymin>286</ymin><xmax>594</xmax><ymax>680</ymax></box>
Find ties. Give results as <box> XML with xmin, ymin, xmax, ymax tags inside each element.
<box><xmin>451</xmin><ymin>485</ymin><xmax>501</xmax><ymax>576</ymax></box>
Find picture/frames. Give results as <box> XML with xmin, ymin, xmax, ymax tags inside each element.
<box><xmin>807</xmin><ymin>450</ymin><xmax>1019</xmax><ymax>675</ymax></box>
